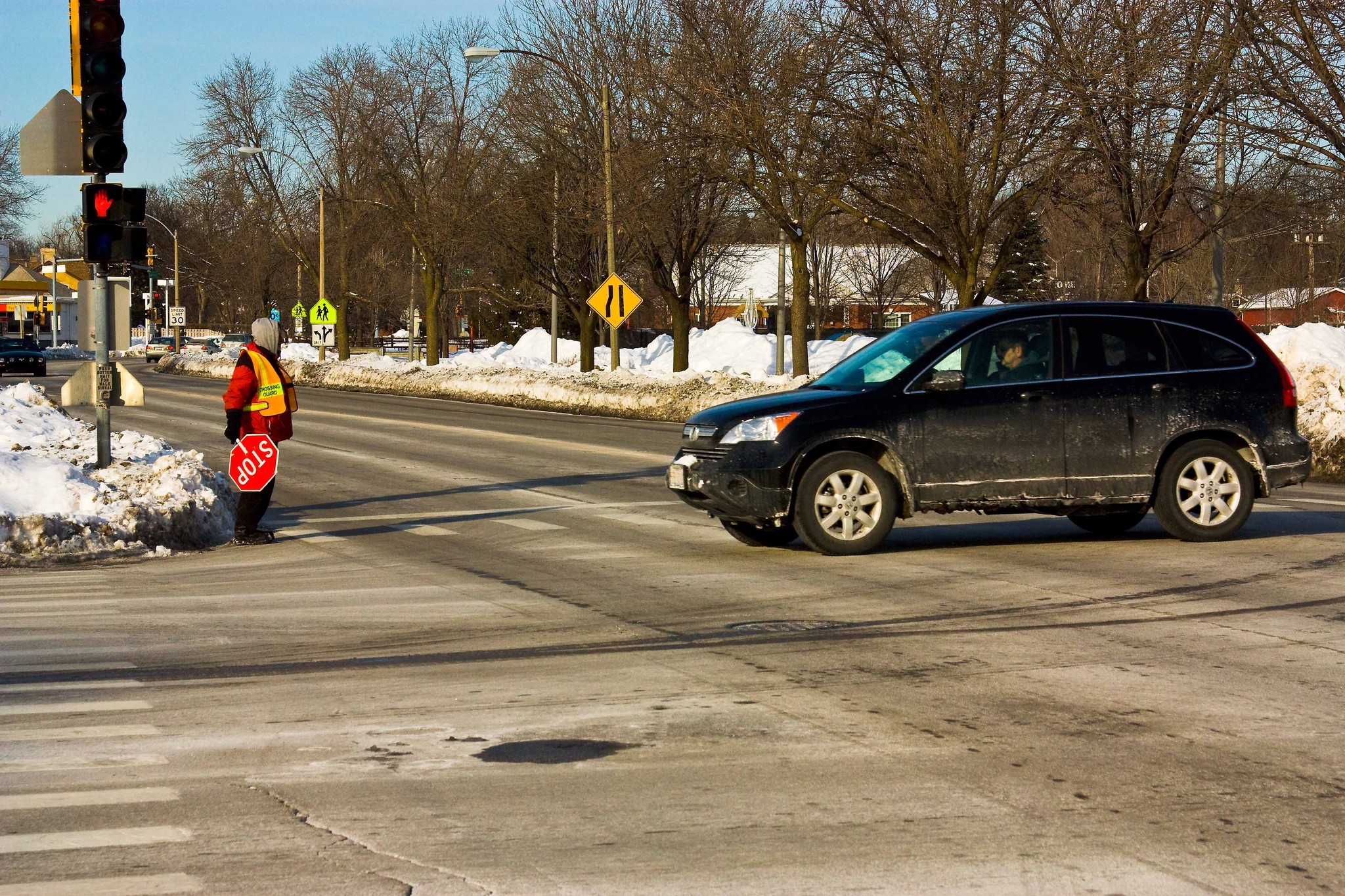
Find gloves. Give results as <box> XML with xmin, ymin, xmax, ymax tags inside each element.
<box><xmin>224</xmin><ymin>407</ymin><xmax>243</xmax><ymax>446</ymax></box>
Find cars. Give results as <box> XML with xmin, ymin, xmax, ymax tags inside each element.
<box><xmin>146</xmin><ymin>333</ymin><xmax>253</xmax><ymax>363</ymax></box>
<box><xmin>0</xmin><ymin>338</ymin><xmax>47</xmax><ymax>376</ymax></box>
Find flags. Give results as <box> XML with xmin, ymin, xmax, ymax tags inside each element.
<box><xmin>14</xmin><ymin>305</ymin><xmax>21</xmax><ymax>320</ymax></box>
<box><xmin>21</xmin><ymin>305</ymin><xmax>28</xmax><ymax>320</ymax></box>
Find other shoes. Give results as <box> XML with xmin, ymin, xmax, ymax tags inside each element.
<box><xmin>233</xmin><ymin>528</ymin><xmax>275</xmax><ymax>544</ymax></box>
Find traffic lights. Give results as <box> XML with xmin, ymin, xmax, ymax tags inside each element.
<box><xmin>145</xmin><ymin>310</ymin><xmax>151</xmax><ymax>319</ymax></box>
<box><xmin>34</xmin><ymin>314</ymin><xmax>40</xmax><ymax>325</ymax></box>
<box><xmin>83</xmin><ymin>183</ymin><xmax>148</xmax><ymax>260</ymax></box>
<box><xmin>40</xmin><ymin>295</ymin><xmax>48</xmax><ymax>313</ymax></box>
<box><xmin>152</xmin><ymin>292</ymin><xmax>159</xmax><ymax>298</ymax></box>
<box><xmin>76</xmin><ymin>0</ymin><xmax>128</xmax><ymax>171</ymax></box>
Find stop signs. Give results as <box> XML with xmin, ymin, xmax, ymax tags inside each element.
<box><xmin>229</xmin><ymin>433</ymin><xmax>279</xmax><ymax>492</ymax></box>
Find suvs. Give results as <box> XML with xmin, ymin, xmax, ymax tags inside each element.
<box><xmin>667</xmin><ymin>298</ymin><xmax>1312</xmax><ymax>553</ymax></box>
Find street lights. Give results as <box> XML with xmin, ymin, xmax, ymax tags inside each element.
<box><xmin>237</xmin><ymin>147</ymin><xmax>326</xmax><ymax>362</ymax></box>
<box><xmin>464</xmin><ymin>46</ymin><xmax>623</xmax><ymax>372</ymax></box>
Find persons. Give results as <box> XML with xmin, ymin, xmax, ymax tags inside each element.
<box><xmin>222</xmin><ymin>316</ymin><xmax>299</xmax><ymax>547</ymax></box>
<box><xmin>961</xmin><ymin>328</ymin><xmax>1049</xmax><ymax>387</ymax></box>
